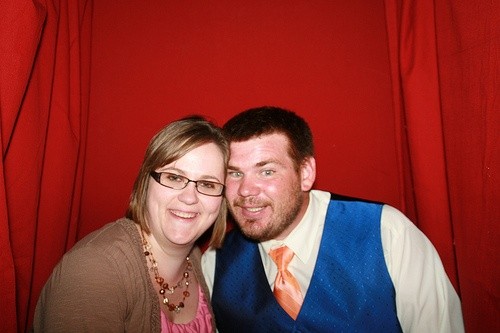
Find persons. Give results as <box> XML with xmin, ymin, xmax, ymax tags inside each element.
<box><xmin>33</xmin><ymin>114</ymin><xmax>231</xmax><ymax>333</ymax></box>
<box><xmin>201</xmin><ymin>105</ymin><xmax>467</xmax><ymax>333</ymax></box>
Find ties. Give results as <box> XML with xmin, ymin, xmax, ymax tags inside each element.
<box><xmin>268</xmin><ymin>245</ymin><xmax>303</xmax><ymax>320</ymax></box>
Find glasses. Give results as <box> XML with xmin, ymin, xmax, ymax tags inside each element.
<box><xmin>150</xmin><ymin>170</ymin><xmax>225</xmax><ymax>197</ymax></box>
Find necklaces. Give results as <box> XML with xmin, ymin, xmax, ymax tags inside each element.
<box><xmin>140</xmin><ymin>227</ymin><xmax>194</xmax><ymax>314</ymax></box>
